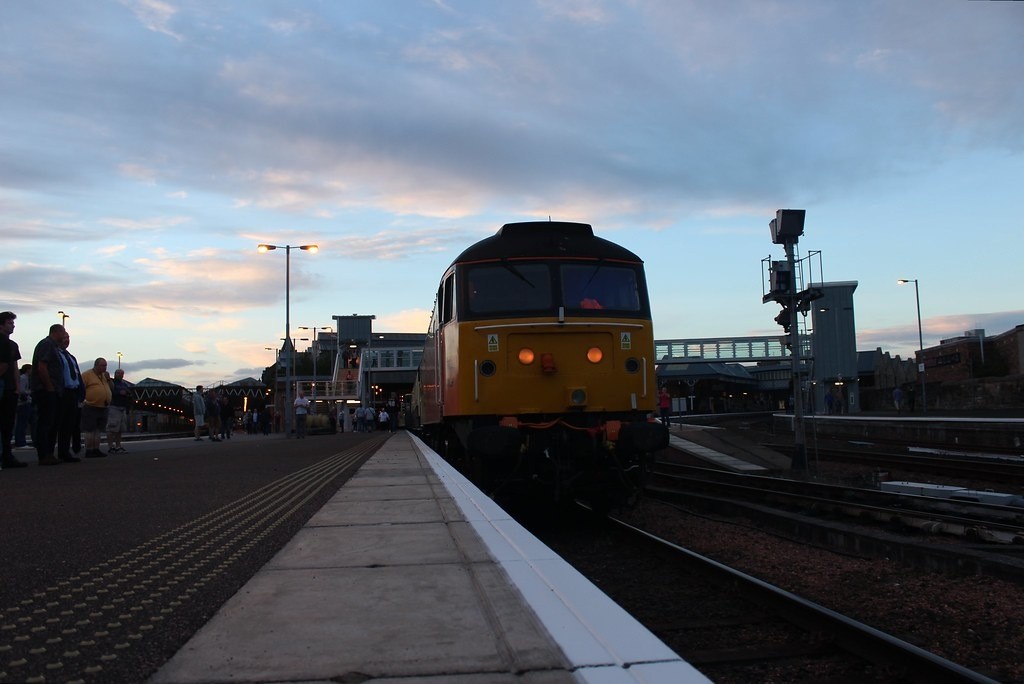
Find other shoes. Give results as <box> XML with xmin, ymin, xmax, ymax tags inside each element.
<box><xmin>2</xmin><ymin>459</ymin><xmax>28</xmax><ymax>468</ymax></box>
<box><xmin>216</xmin><ymin>438</ymin><xmax>221</xmax><ymax>441</ymax></box>
<box><xmin>94</xmin><ymin>449</ymin><xmax>107</xmax><ymax>456</ymax></box>
<box><xmin>212</xmin><ymin>437</ymin><xmax>216</xmax><ymax>441</ymax></box>
<box><xmin>85</xmin><ymin>450</ymin><xmax>97</xmax><ymax>457</ymax></box>
<box><xmin>194</xmin><ymin>437</ymin><xmax>202</xmax><ymax>440</ymax></box>
<box><xmin>72</xmin><ymin>453</ymin><xmax>80</xmax><ymax>458</ymax></box>
<box><xmin>58</xmin><ymin>454</ymin><xmax>81</xmax><ymax>462</ymax></box>
<box><xmin>39</xmin><ymin>455</ymin><xmax>62</xmax><ymax>464</ymax></box>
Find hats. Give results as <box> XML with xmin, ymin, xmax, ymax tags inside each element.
<box><xmin>196</xmin><ymin>386</ymin><xmax>205</xmax><ymax>389</ymax></box>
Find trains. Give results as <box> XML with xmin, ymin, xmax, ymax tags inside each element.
<box><xmin>409</xmin><ymin>220</ymin><xmax>669</xmax><ymax>512</ymax></box>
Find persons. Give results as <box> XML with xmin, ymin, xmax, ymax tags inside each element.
<box><xmin>191</xmin><ymin>349</ymin><xmax>404</xmax><ymax>442</ymax></box>
<box><xmin>784</xmin><ymin>395</ymin><xmax>794</xmax><ymax>415</ymax></box>
<box><xmin>892</xmin><ymin>385</ymin><xmax>916</xmax><ymax>416</ymax></box>
<box><xmin>659</xmin><ymin>387</ymin><xmax>671</xmax><ymax>428</ymax></box>
<box><xmin>824</xmin><ymin>389</ymin><xmax>841</xmax><ymax>415</ymax></box>
<box><xmin>0</xmin><ymin>312</ymin><xmax>130</xmax><ymax>470</ymax></box>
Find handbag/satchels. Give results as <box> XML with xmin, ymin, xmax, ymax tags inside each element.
<box><xmin>373</xmin><ymin>413</ymin><xmax>378</xmax><ymax>420</ymax></box>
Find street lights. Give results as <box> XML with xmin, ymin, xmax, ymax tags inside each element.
<box><xmin>117</xmin><ymin>351</ymin><xmax>123</xmax><ymax>370</ymax></box>
<box><xmin>280</xmin><ymin>337</ymin><xmax>309</xmax><ymax>434</ymax></box>
<box><xmin>58</xmin><ymin>310</ymin><xmax>69</xmax><ymax>326</ymax></box>
<box><xmin>896</xmin><ymin>278</ymin><xmax>928</xmax><ymax>417</ymax></box>
<box><xmin>256</xmin><ymin>242</ymin><xmax>318</xmax><ymax>438</ymax></box>
<box><xmin>301</xmin><ymin>327</ymin><xmax>329</xmax><ymax>434</ymax></box>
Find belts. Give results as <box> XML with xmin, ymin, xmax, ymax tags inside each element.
<box><xmin>62</xmin><ymin>351</ymin><xmax>77</xmax><ymax>381</ymax></box>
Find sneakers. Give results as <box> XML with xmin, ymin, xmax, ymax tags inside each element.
<box><xmin>119</xmin><ymin>448</ymin><xmax>129</xmax><ymax>453</ymax></box>
<box><xmin>108</xmin><ymin>447</ymin><xmax>124</xmax><ymax>454</ymax></box>
